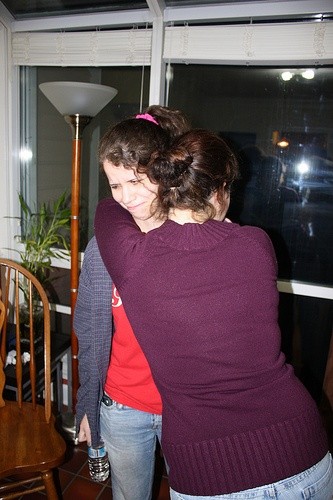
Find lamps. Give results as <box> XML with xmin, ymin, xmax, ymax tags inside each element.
<box><xmin>39</xmin><ymin>81</ymin><xmax>118</xmax><ymax>445</ymax></box>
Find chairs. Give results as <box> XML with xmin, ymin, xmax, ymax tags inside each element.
<box><xmin>0</xmin><ymin>259</ymin><xmax>66</xmax><ymax>500</ymax></box>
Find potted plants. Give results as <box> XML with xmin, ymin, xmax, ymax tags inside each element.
<box><xmin>5</xmin><ymin>189</ymin><xmax>81</xmax><ymax>344</ymax></box>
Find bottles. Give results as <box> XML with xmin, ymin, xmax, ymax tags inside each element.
<box><xmin>89</xmin><ymin>435</ymin><xmax>109</xmax><ymax>482</ymax></box>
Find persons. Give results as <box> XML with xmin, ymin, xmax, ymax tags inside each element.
<box><xmin>71</xmin><ymin>106</ymin><xmax>333</xmax><ymax>500</ymax></box>
